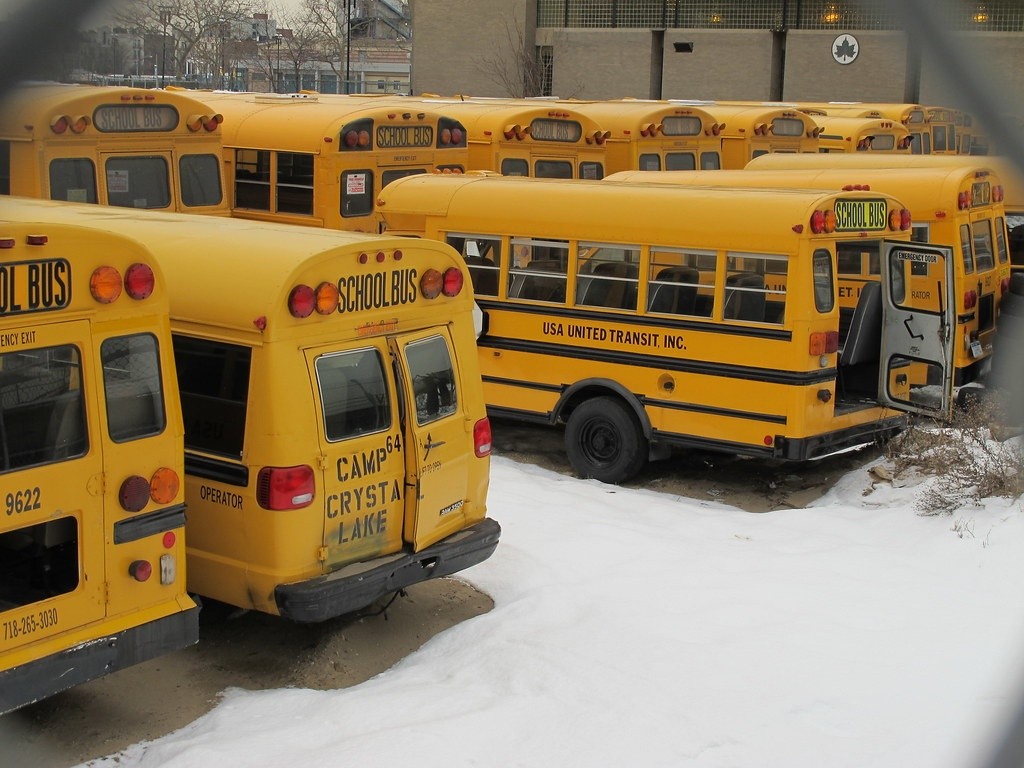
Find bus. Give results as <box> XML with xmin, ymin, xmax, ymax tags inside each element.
<box><xmin>1</xmin><ymin>192</ymin><xmax>502</xmax><ymax>626</ymax></box>
<box><xmin>0</xmin><ymin>212</ymin><xmax>206</xmax><ymax>727</ymax></box>
<box><xmin>0</xmin><ymin>82</ymin><xmax>1024</xmax><ymax>485</ymax></box>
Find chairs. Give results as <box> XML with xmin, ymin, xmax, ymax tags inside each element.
<box><xmin>237</xmin><ymin>169</ymin><xmax>373</xmax><ymax>215</ymax></box>
<box><xmin>52</xmin><ymin>156</ymin><xmax>219</xmax><ymax>212</ymax></box>
<box><xmin>175</xmin><ymin>340</ymin><xmax>447</xmax><ymax>467</ymax></box>
<box><xmin>471</xmin><ymin>259</ymin><xmax>882</xmax><ymax>391</ymax></box>
<box><xmin>0</xmin><ymin>353</ymin><xmax>157</xmax><ymax>601</ymax></box>
